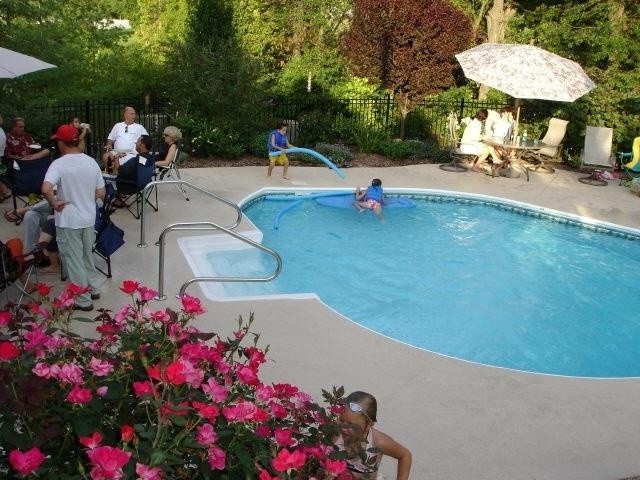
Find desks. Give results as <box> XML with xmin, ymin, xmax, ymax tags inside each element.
<box><xmin>483</xmin><ymin>136</ymin><xmax>550</xmax><ymax>184</ymax></box>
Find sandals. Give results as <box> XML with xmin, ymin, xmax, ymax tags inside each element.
<box><xmin>0</xmin><ymin>190</ymin><xmax>12</xmax><ymax>203</ymax></box>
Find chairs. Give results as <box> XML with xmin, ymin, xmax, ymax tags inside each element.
<box><xmin>437</xmin><ymin>107</ymin><xmax>478</xmax><ymax>175</ymax></box>
<box><xmin>0</xmin><ymin>239</ymin><xmax>50</xmax><ymax>309</ymax></box>
<box><xmin>57</xmin><ymin>190</ymin><xmax>127</xmax><ymax>282</ymax></box>
<box><xmin>616</xmin><ymin>133</ymin><xmax>640</xmax><ymax>189</ymax></box>
<box><xmin>0</xmin><ymin>155</ymin><xmax>53</xmax><ymax>226</ymax></box>
<box><xmin>577</xmin><ymin>123</ymin><xmax>616</xmax><ymax>188</ymax></box>
<box><xmin>527</xmin><ymin>115</ymin><xmax>570</xmax><ymax>175</ymax></box>
<box><xmin>93</xmin><ymin>143</ymin><xmax>159</xmax><ymax>225</ymax></box>
<box><xmin>145</xmin><ymin>143</ymin><xmax>190</xmax><ymax>210</ymax></box>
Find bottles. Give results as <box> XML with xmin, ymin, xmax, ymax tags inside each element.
<box><xmin>516</xmin><ymin>135</ymin><xmax>520</xmax><ymax>144</ymax></box>
<box><xmin>107</xmin><ymin>156</ymin><xmax>114</xmax><ymax>174</ymax></box>
<box><xmin>77</xmin><ymin>122</ymin><xmax>90</xmax><ymax>129</ymax></box>
<box><xmin>523</xmin><ymin>130</ymin><xmax>528</xmax><ymax>143</ymax></box>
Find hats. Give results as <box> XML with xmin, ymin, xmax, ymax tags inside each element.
<box><xmin>51</xmin><ymin>125</ymin><xmax>78</xmax><ymax>143</ymax></box>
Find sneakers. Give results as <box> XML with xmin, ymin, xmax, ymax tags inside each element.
<box><xmin>72</xmin><ymin>303</ymin><xmax>93</xmax><ymax>311</ymax></box>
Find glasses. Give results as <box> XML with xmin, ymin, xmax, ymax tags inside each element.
<box><xmin>349</xmin><ymin>403</ymin><xmax>373</xmax><ymax>425</ymax></box>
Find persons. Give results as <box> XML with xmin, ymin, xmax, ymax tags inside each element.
<box><xmin>96</xmin><ymin>134</ymin><xmax>153</xmax><ymax>209</ymax></box>
<box><xmin>103</xmin><ymin>105</ymin><xmax>150</xmax><ymax>167</ymax></box>
<box><xmin>265</xmin><ymin>123</ymin><xmax>298</xmax><ymax>182</ymax></box>
<box><xmin>65</xmin><ymin>116</ymin><xmax>92</xmax><ymax>143</ymax></box>
<box><xmin>351</xmin><ymin>179</ymin><xmax>386</xmax><ymax>225</ymax></box>
<box><xmin>151</xmin><ymin>121</ymin><xmax>184</xmax><ymax>171</ymax></box>
<box><xmin>0</xmin><ymin>115</ymin><xmax>61</xmax><ymax>297</ymax></box>
<box><xmin>492</xmin><ymin>106</ymin><xmax>516</xmax><ymax>138</ymax></box>
<box><xmin>40</xmin><ymin>124</ymin><xmax>107</xmax><ymax>311</ymax></box>
<box><xmin>458</xmin><ymin>109</ymin><xmax>507</xmax><ymax>174</ymax></box>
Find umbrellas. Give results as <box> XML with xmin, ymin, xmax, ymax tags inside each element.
<box><xmin>454</xmin><ymin>42</ymin><xmax>597</xmax><ymax>179</ymax></box>
<box><xmin>0</xmin><ymin>46</ymin><xmax>58</xmax><ymax>79</ymax></box>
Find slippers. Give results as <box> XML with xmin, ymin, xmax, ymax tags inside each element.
<box><xmin>5</xmin><ymin>209</ymin><xmax>23</xmax><ymax>222</ymax></box>
